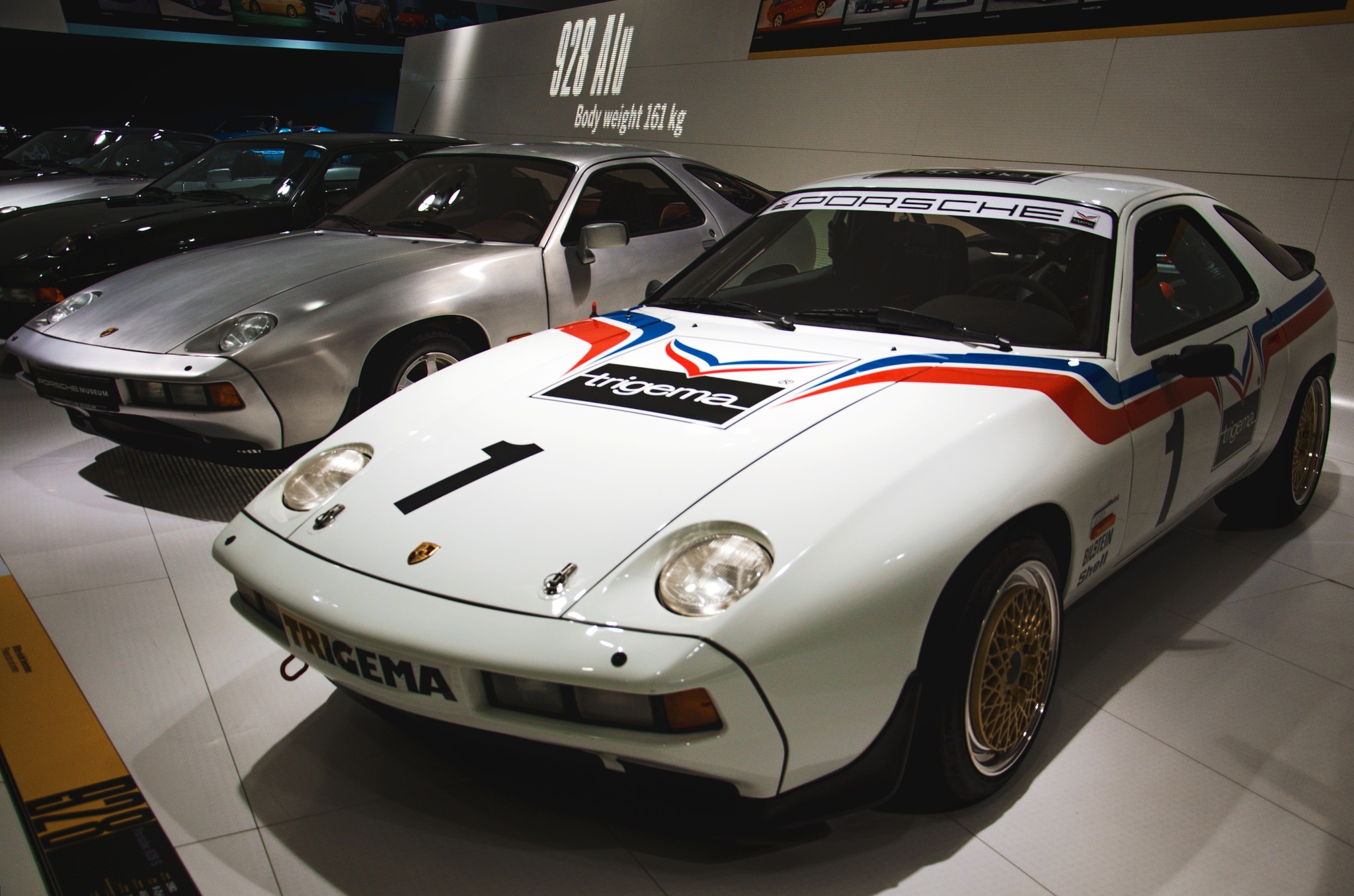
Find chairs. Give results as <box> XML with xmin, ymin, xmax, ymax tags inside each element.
<box><xmin>861</xmin><ymin>223</ymin><xmax>972</xmax><ymax>314</ymax></box>
<box><xmin>571</xmin><ymin>195</ymin><xmax>602</xmax><ymax>222</ymax></box>
<box><xmin>656</xmin><ymin>202</ymin><xmax>695</xmax><ymax>229</ymax></box>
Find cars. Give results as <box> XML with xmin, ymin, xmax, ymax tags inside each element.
<box><xmin>853</xmin><ymin>1</ymin><xmax>910</xmax><ymax>13</ymax></box>
<box><xmin>765</xmin><ymin>0</ymin><xmax>838</xmax><ymax>25</ymax></box>
<box><xmin>175</xmin><ymin>0</ymin><xmax>224</xmax><ymax>13</ymax></box>
<box><xmin>0</xmin><ymin>127</ymin><xmax>219</xmax><ymax>216</ymax></box>
<box><xmin>209</xmin><ymin>114</ymin><xmax>338</xmax><ymax>139</ymax></box>
<box><xmin>393</xmin><ymin>5</ymin><xmax>428</xmax><ymax>36</ymax></box>
<box><xmin>353</xmin><ymin>0</ymin><xmax>389</xmax><ymax>27</ymax></box>
<box><xmin>312</xmin><ymin>0</ymin><xmax>349</xmax><ymax>25</ymax></box>
<box><xmin>434</xmin><ymin>9</ymin><xmax>474</xmax><ymax>31</ymax></box>
<box><xmin>241</xmin><ymin>0</ymin><xmax>307</xmax><ymax>18</ymax></box>
<box><xmin>207</xmin><ymin>168</ymin><xmax>1337</xmax><ymax>827</ymax></box>
<box><xmin>0</xmin><ymin>80</ymin><xmax>480</xmax><ymax>381</ymax></box>
<box><xmin>12</xmin><ymin>140</ymin><xmax>781</xmax><ymax>470</ymax></box>
<box><xmin>2</xmin><ymin>127</ymin><xmax>120</xmax><ymax>178</ymax></box>
